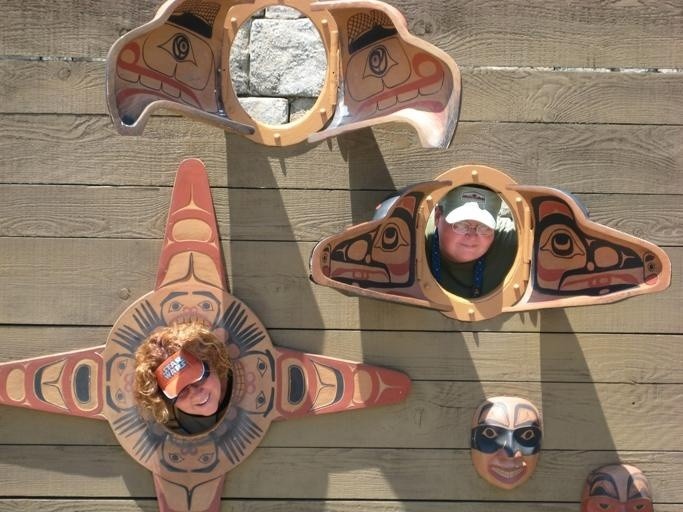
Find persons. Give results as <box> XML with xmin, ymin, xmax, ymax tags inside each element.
<box><xmin>424</xmin><ymin>184</ymin><xmax>518</xmax><ymax>301</ymax></box>
<box><xmin>131</xmin><ymin>324</ymin><xmax>232</xmax><ymax>436</ymax></box>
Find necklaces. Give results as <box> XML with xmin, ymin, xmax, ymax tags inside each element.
<box><xmin>432</xmin><ymin>228</ymin><xmax>486</xmax><ymax>298</ymax></box>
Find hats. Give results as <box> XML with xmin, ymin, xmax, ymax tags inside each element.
<box><xmin>153</xmin><ymin>347</ymin><xmax>208</xmax><ymax>400</ymax></box>
<box><xmin>437</xmin><ymin>182</ymin><xmax>502</xmax><ymax>231</ymax></box>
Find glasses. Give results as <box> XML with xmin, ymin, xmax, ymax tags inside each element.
<box><xmin>172</xmin><ymin>361</ymin><xmax>212</xmax><ymax>401</ymax></box>
<box><xmin>450</xmin><ymin>221</ymin><xmax>492</xmax><ymax>237</ymax></box>
